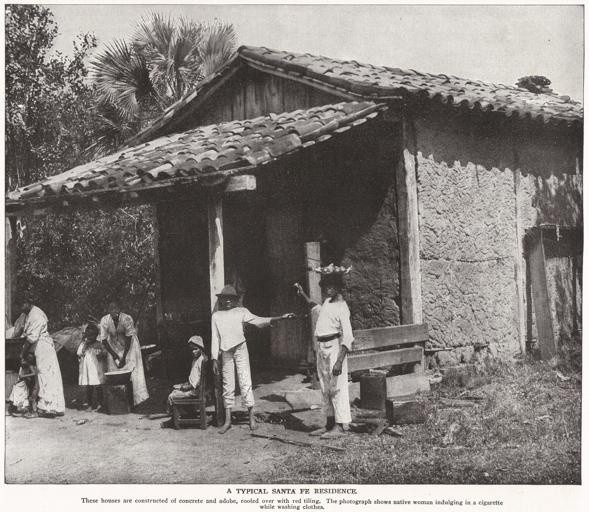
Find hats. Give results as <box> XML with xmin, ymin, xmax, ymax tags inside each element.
<box><xmin>188</xmin><ymin>335</ymin><xmax>204</xmax><ymax>348</ymax></box>
<box><xmin>216</xmin><ymin>286</ymin><xmax>237</xmax><ymax>296</ymax></box>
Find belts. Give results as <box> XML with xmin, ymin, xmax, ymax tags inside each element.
<box><xmin>318</xmin><ymin>334</ymin><xmax>339</xmax><ymax>342</ymax></box>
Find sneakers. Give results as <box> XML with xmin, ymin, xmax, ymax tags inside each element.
<box><xmin>310</xmin><ymin>428</ymin><xmax>348</xmax><ymax>439</ymax></box>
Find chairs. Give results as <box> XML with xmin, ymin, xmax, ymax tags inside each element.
<box><xmin>169</xmin><ymin>359</ymin><xmax>208</xmax><ymax>432</ymax></box>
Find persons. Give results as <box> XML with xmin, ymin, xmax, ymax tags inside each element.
<box><xmin>295</xmin><ymin>272</ymin><xmax>352</xmax><ymax>438</ymax></box>
<box><xmin>8</xmin><ymin>352</ymin><xmax>36</xmax><ymax>417</ymax></box>
<box><xmin>99</xmin><ymin>301</ymin><xmax>150</xmax><ymax>407</ymax></box>
<box><xmin>210</xmin><ymin>284</ymin><xmax>297</xmax><ymax>433</ymax></box>
<box><xmin>76</xmin><ymin>324</ymin><xmax>107</xmax><ymax>410</ymax></box>
<box><xmin>12</xmin><ymin>295</ymin><xmax>66</xmax><ymax>417</ymax></box>
<box><xmin>160</xmin><ymin>335</ymin><xmax>209</xmax><ymax>428</ymax></box>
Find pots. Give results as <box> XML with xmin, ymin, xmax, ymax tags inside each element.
<box><xmin>104</xmin><ymin>370</ymin><xmax>132</xmax><ymax>385</ymax></box>
